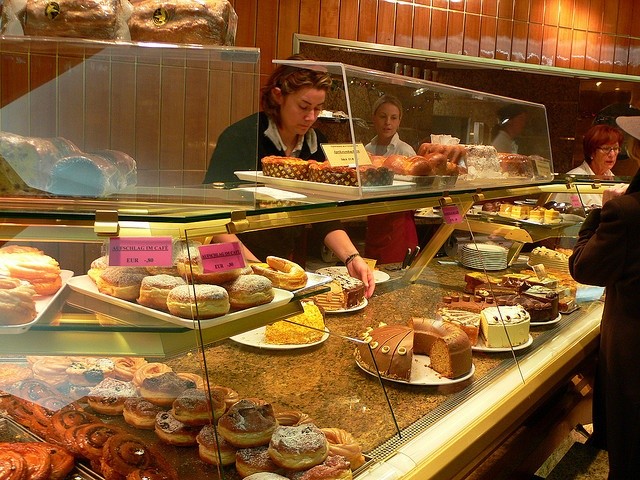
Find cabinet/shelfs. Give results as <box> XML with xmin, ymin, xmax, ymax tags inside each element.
<box><xmin>0</xmin><ymin>182</ymin><xmax>629</xmax><ymax>480</ymax></box>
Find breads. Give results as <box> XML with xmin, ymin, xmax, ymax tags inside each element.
<box><xmin>204</xmin><ymin>382</ymin><xmax>238</xmax><ymax>407</ymax></box>
<box><xmin>2</xmin><ymin>245</ymin><xmax>45</xmax><ymax>258</ymax></box>
<box><xmin>198</xmin><ymin>425</ymin><xmax>235</xmax><ymax>467</ymax></box>
<box><xmin>137</xmin><ymin>274</ymin><xmax>186</xmax><ymax>308</ymax></box>
<box><xmin>125</xmin><ymin>396</ymin><xmax>160</xmax><ymax>428</ymax></box>
<box><xmin>32</xmin><ymin>357</ymin><xmax>66</xmax><ymax>385</ymax></box>
<box><xmin>77</xmin><ymin>420</ymin><xmax>125</xmax><ymax>460</ymax></box>
<box><xmin>52</xmin><ymin>149</ymin><xmax>137</xmax><ymax>198</ymax></box>
<box><xmin>89</xmin><ymin>377</ymin><xmax>134</xmax><ymax>417</ymax></box>
<box><xmin>0</xmin><ymin>363</ymin><xmax>31</xmax><ymax>386</ymax></box>
<box><xmin>169</xmin><ymin>284</ymin><xmax>230</xmax><ymax>319</ymax></box>
<box><xmin>173</xmin><ymin>390</ymin><xmax>222</xmax><ymax>424</ymax></box>
<box><xmin>89</xmin><ymin>256</ymin><xmax>116</xmax><ymax>273</ymax></box>
<box><xmin>369</xmin><ymin>151</ymin><xmax>458</xmax><ymax>184</ymax></box>
<box><xmin>255</xmin><ymin>255</ymin><xmax>307</xmax><ymax>289</ymax></box>
<box><xmin>236</xmin><ymin>448</ymin><xmax>279</xmax><ymax>475</ymax></box>
<box><xmin>35</xmin><ymin>395</ymin><xmax>79</xmax><ymax>425</ymax></box>
<box><xmin>1</xmin><ymin>131</ymin><xmax>83</xmax><ymax>197</ymax></box>
<box><xmin>0</xmin><ymin>439</ymin><xmax>73</xmax><ymax>480</ymax></box>
<box><xmin>147</xmin><ymin>242</ymin><xmax>200</xmax><ymax>275</ymax></box>
<box><xmin>275</xmin><ymin>409</ymin><xmax>311</xmax><ymax>424</ymax></box>
<box><xmin>179</xmin><ymin>366</ymin><xmax>207</xmax><ymax>390</ymax></box>
<box><xmin>321</xmin><ymin>428</ymin><xmax>363</xmax><ymax>469</ymax></box>
<box><xmin>67</xmin><ymin>425</ymin><xmax>100</xmax><ymax>454</ymax></box>
<box><xmin>19</xmin><ymin>0</ymin><xmax>128</xmax><ymax>40</ymax></box>
<box><xmin>216</xmin><ymin>399</ymin><xmax>276</xmax><ymax>446</ymax></box>
<box><xmin>141</xmin><ymin>371</ymin><xmax>196</xmax><ymax>404</ymax></box>
<box><xmin>129</xmin><ymin>451</ymin><xmax>179</xmax><ymax>479</ymax></box>
<box><xmin>288</xmin><ymin>456</ymin><xmax>352</xmax><ymax>480</ymax></box>
<box><xmin>104</xmin><ymin>435</ymin><xmax>154</xmax><ymax>477</ymax></box>
<box><xmin>113</xmin><ymin>354</ymin><xmax>142</xmax><ymax>376</ymax></box>
<box><xmin>269</xmin><ymin>423</ymin><xmax>328</xmax><ymax>468</ymax></box>
<box><xmin>228</xmin><ymin>277</ymin><xmax>273</xmax><ymax>306</ymax></box>
<box><xmin>262</xmin><ymin>154</ymin><xmax>304</xmax><ymax>178</ymax></box>
<box><xmin>132</xmin><ymin>364</ymin><xmax>170</xmax><ymax>384</ymax></box>
<box><xmin>13</xmin><ymin>380</ymin><xmax>59</xmax><ymax>410</ymax></box>
<box><xmin>52</xmin><ymin>410</ymin><xmax>103</xmax><ymax>440</ymax></box>
<box><xmin>97</xmin><ymin>265</ymin><xmax>147</xmax><ymax>299</ymax></box>
<box><xmin>68</xmin><ymin>364</ymin><xmax>105</xmax><ymax>404</ymax></box>
<box><xmin>3</xmin><ymin>254</ymin><xmax>62</xmax><ymax>295</ymax></box>
<box><xmin>174</xmin><ymin>248</ymin><xmax>254</xmax><ymax>284</ymax></box>
<box><xmin>156</xmin><ymin>410</ymin><xmax>198</xmax><ymax>445</ymax></box>
<box><xmin>306</xmin><ymin>159</ymin><xmax>392</xmax><ymax>187</ymax></box>
<box><xmin>0</xmin><ymin>280</ymin><xmax>36</xmax><ymax>328</ymax></box>
<box><xmin>129</xmin><ymin>0</ymin><xmax>239</xmax><ymax>44</ymax></box>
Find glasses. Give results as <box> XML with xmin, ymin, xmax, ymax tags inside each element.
<box><xmin>597</xmin><ymin>146</ymin><xmax>619</xmax><ymax>153</ymax></box>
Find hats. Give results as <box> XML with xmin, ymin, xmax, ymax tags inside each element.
<box><xmin>616</xmin><ymin>116</ymin><xmax>639</xmax><ymax>139</ymax></box>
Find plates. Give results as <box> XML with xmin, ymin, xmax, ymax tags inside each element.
<box><xmin>471</xmin><ymin>332</ymin><xmax>534</xmax><ymax>355</ymax></box>
<box><xmin>292</xmin><ymin>271</ymin><xmax>334</xmax><ymax>297</ymax></box>
<box><xmin>431</xmin><ymin>173</ymin><xmax>458</xmax><ymax>189</ymax></box>
<box><xmin>459</xmin><ymin>243</ymin><xmax>512</xmax><ymax>273</ymax></box>
<box><xmin>527</xmin><ymin>249</ymin><xmax>574</xmax><ymax>271</ymax></box>
<box><xmin>317</xmin><ymin>264</ymin><xmax>391</xmax><ymax>287</ymax></box>
<box><xmin>64</xmin><ymin>274</ymin><xmax>293</xmax><ymax>332</ymax></box>
<box><xmin>529</xmin><ymin>312</ymin><xmax>563</xmax><ymax>327</ymax></box>
<box><xmin>2</xmin><ymin>414</ymin><xmax>98</xmax><ymax>480</ymax></box>
<box><xmin>355</xmin><ymin>338</ymin><xmax>477</xmax><ymax>392</ymax></box>
<box><xmin>233</xmin><ymin>167</ymin><xmax>416</xmax><ymax>198</ymax></box>
<box><xmin>394</xmin><ymin>167</ymin><xmax>435</xmax><ymax>190</ymax></box>
<box><xmin>301</xmin><ymin>297</ymin><xmax>369</xmax><ymax>315</ymax></box>
<box><xmin>7</xmin><ymin>412</ymin><xmax>106</xmax><ymax>479</ymax></box>
<box><xmin>0</xmin><ymin>269</ymin><xmax>73</xmax><ymax>334</ymax></box>
<box><xmin>230</xmin><ymin>325</ymin><xmax>330</xmax><ymax>353</ymax></box>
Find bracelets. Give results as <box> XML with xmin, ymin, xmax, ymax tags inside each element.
<box><xmin>340</xmin><ymin>252</ymin><xmax>362</xmax><ymax>265</ymax></box>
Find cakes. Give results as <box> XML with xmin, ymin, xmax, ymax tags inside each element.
<box><xmin>311</xmin><ymin>273</ymin><xmax>365</xmax><ymax>309</ymax></box>
<box><xmin>441</xmin><ymin>295</ymin><xmax>513</xmax><ymax>315</ymax></box>
<box><xmin>419</xmin><ymin>132</ymin><xmax>502</xmax><ymax>179</ymax></box>
<box><xmin>501</xmin><ymin>153</ymin><xmax>552</xmax><ymax>178</ymax></box>
<box><xmin>509</xmin><ymin>206</ymin><xmax>527</xmax><ymax>220</ymax></box>
<box><xmin>510</xmin><ymin>293</ymin><xmax>552</xmax><ymax>322</ymax></box>
<box><xmin>506</xmin><ymin>274</ymin><xmax>576</xmax><ymax>312</ymax></box>
<box><xmin>528</xmin><ymin>208</ymin><xmax>543</xmax><ymax>224</ymax></box>
<box><xmin>464</xmin><ymin>272</ymin><xmax>499</xmax><ymax>288</ymax></box>
<box><xmin>500</xmin><ymin>202</ymin><xmax>512</xmax><ymax>216</ymax></box>
<box><xmin>482</xmin><ymin>306</ymin><xmax>529</xmax><ymax>347</ymax></box>
<box><xmin>523</xmin><ymin>284</ymin><xmax>559</xmax><ymax>320</ymax></box>
<box><xmin>411</xmin><ymin>315</ymin><xmax>472</xmax><ymax>378</ymax></box>
<box><xmin>474</xmin><ymin>280</ymin><xmax>523</xmax><ymax>303</ymax></box>
<box><xmin>356</xmin><ymin>323</ymin><xmax>413</xmax><ymax>382</ymax></box>
<box><xmin>441</xmin><ymin>309</ymin><xmax>483</xmax><ymax>346</ymax></box>
<box><xmin>268</xmin><ymin>302</ymin><xmax>324</xmax><ymax>346</ymax></box>
<box><xmin>529</xmin><ymin>246</ymin><xmax>571</xmax><ymax>273</ymax></box>
<box><xmin>545</xmin><ymin>210</ymin><xmax>559</xmax><ymax>225</ymax></box>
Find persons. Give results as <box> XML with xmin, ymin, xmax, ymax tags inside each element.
<box><xmin>553</xmin><ymin>122</ymin><xmax>629</xmax><ymax>250</ymax></box>
<box><xmin>363</xmin><ymin>94</ymin><xmax>420</xmax><ymax>265</ymax></box>
<box><xmin>490</xmin><ymin>101</ymin><xmax>532</xmax><ymax>174</ymax></box>
<box><xmin>568</xmin><ymin>115</ymin><xmax>640</xmax><ymax>472</ymax></box>
<box><xmin>202</xmin><ymin>54</ymin><xmax>376</xmax><ymax>297</ymax></box>
